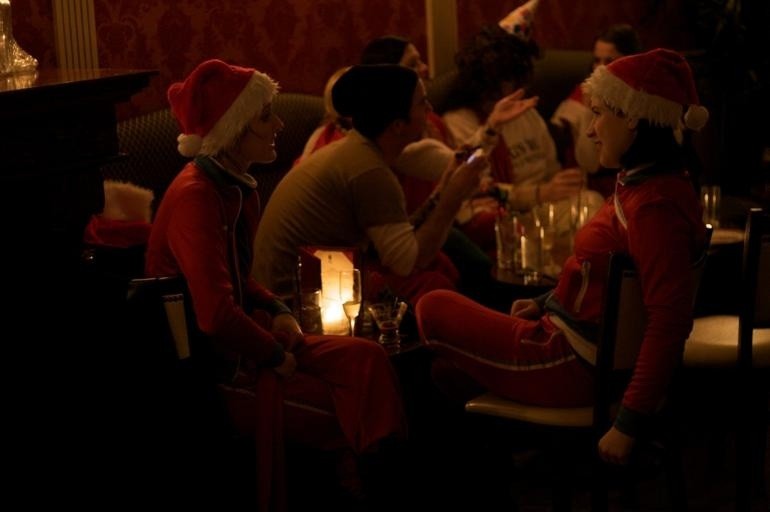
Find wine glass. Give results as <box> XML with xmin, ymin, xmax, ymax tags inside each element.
<box><xmin>338</xmin><ymin>268</ymin><xmax>361</xmax><ymax>339</ymax></box>
<box><xmin>366</xmin><ymin>301</ymin><xmax>408</xmax><ymax>345</ymax></box>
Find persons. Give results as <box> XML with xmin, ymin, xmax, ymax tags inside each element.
<box><xmin>251</xmin><ymin>1</ymin><xmax>769</xmax><ymax>344</ymax></box>
<box><xmin>415</xmin><ymin>46</ymin><xmax>710</xmax><ymax>464</ymax></box>
<box><xmin>144</xmin><ymin>58</ymin><xmax>413</xmax><ymax>511</ymax></box>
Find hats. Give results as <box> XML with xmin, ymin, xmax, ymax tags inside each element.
<box><xmin>167</xmin><ymin>59</ymin><xmax>281</xmax><ymax>156</ymax></box>
<box><xmin>580</xmin><ymin>48</ymin><xmax>709</xmax><ymax>128</ymax></box>
<box><xmin>497</xmin><ymin>0</ymin><xmax>539</xmax><ymax>41</ymax></box>
<box><xmin>324</xmin><ymin>37</ymin><xmax>418</xmax><ymax>128</ymax></box>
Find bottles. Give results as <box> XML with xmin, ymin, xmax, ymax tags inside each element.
<box><xmin>290</xmin><ymin>255</ymin><xmax>320</xmax><ymax>335</ymax></box>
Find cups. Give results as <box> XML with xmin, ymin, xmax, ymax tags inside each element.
<box><xmin>296</xmin><ymin>287</ymin><xmax>320</xmax><ymax>306</ymax></box>
<box><xmin>491</xmin><ymin>190</ymin><xmax>590</xmax><ymax>281</ymax></box>
<box><xmin>699</xmin><ymin>185</ymin><xmax>721</xmax><ymax>228</ymax></box>
